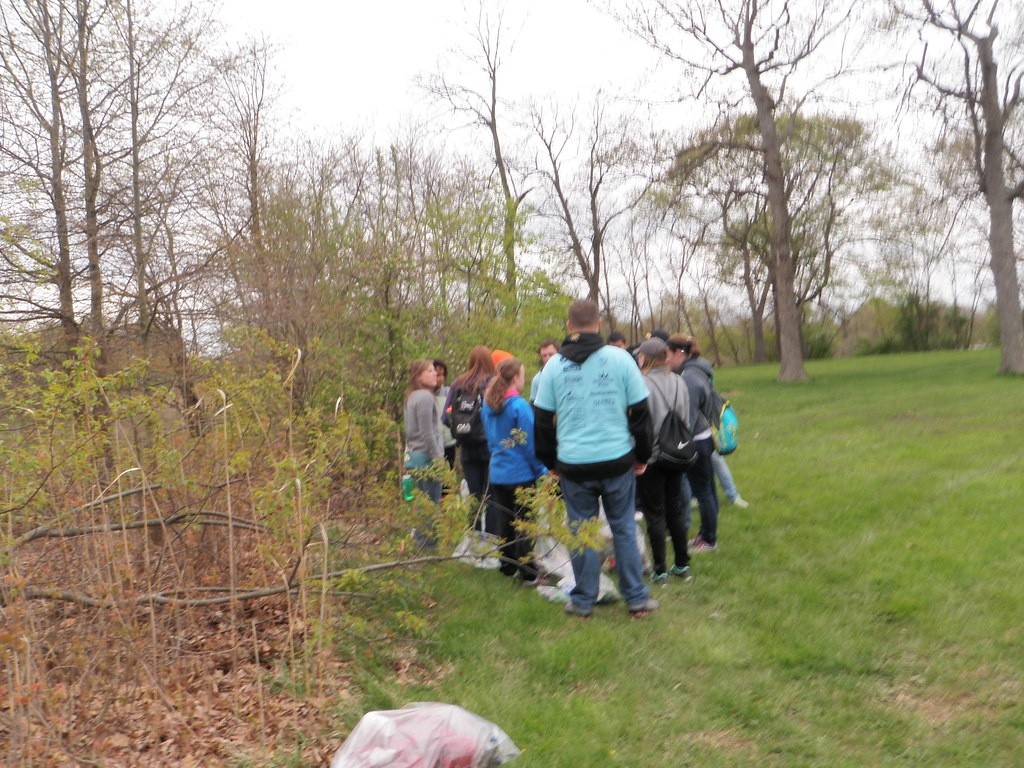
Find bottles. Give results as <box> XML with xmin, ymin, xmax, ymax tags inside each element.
<box><xmin>402</xmin><ymin>471</ymin><xmax>415</xmax><ymax>501</ymax></box>
<box><xmin>446</xmin><ymin>405</ymin><xmax>453</xmax><ymax>422</ymax></box>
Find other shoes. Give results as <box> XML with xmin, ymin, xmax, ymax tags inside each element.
<box><xmin>731</xmin><ymin>497</ymin><xmax>748</xmax><ymax>510</ymax></box>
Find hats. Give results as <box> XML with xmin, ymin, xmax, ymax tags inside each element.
<box><xmin>631</xmin><ymin>336</ymin><xmax>669</xmax><ymax>359</ymax></box>
<box><xmin>491</xmin><ymin>349</ymin><xmax>513</xmax><ymax>365</ymax></box>
<box><xmin>651</xmin><ymin>329</ymin><xmax>671</xmax><ymax>341</ymax></box>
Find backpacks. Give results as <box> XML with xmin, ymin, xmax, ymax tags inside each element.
<box><xmin>645</xmin><ymin>372</ymin><xmax>699</xmax><ymax>473</ymax></box>
<box><xmin>451</xmin><ymin>373</ymin><xmax>495</xmax><ymax>447</ymax></box>
<box><xmin>681</xmin><ymin>362</ymin><xmax>739</xmax><ymax>455</ymax></box>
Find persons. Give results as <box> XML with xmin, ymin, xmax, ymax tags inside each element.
<box><xmin>406</xmin><ymin>328</ymin><xmax>751</xmax><ymax>588</ymax></box>
<box><xmin>532</xmin><ymin>299</ymin><xmax>664</xmax><ymax>622</ymax></box>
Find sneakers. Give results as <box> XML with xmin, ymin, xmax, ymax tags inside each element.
<box><xmin>687</xmin><ymin>533</ymin><xmax>719</xmax><ymax>555</ymax></box>
<box><xmin>667</xmin><ymin>563</ymin><xmax>693</xmax><ymax>582</ymax></box>
<box><xmin>629</xmin><ymin>597</ymin><xmax>659</xmax><ymax>619</ymax></box>
<box><xmin>648</xmin><ymin>570</ymin><xmax>669</xmax><ymax>588</ymax></box>
<box><xmin>561</xmin><ymin>601</ymin><xmax>593</xmax><ymax>616</ymax></box>
